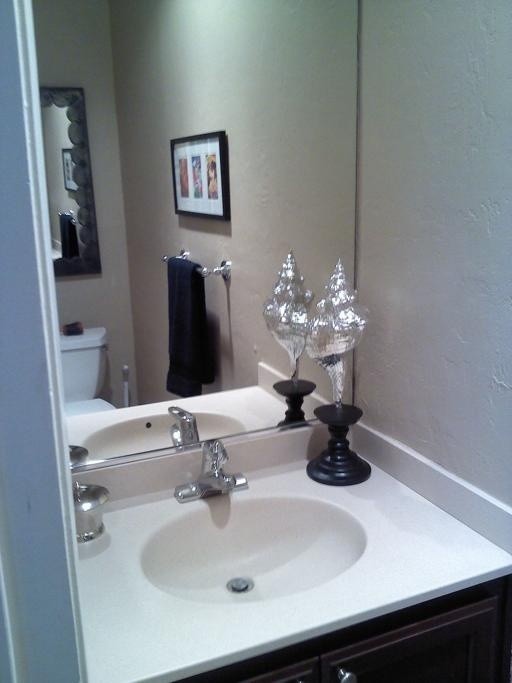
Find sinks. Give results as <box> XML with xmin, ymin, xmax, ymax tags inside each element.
<box><xmin>83</xmin><ymin>412</ymin><xmax>247</xmax><ymax>464</ymax></box>
<box><xmin>138</xmin><ymin>498</ymin><xmax>366</xmax><ymax>603</ymax></box>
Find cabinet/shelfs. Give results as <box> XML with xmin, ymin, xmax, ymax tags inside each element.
<box><xmin>175</xmin><ymin>576</ymin><xmax>511</xmax><ymax>682</ymax></box>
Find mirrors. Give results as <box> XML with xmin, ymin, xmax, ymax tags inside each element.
<box><xmin>39</xmin><ymin>86</ymin><xmax>102</xmax><ymax>280</ymax></box>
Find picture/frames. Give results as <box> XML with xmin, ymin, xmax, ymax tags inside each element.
<box><xmin>62</xmin><ymin>149</ymin><xmax>78</xmax><ymax>192</ymax></box>
<box><xmin>170</xmin><ymin>130</ymin><xmax>230</xmax><ymax>219</ymax></box>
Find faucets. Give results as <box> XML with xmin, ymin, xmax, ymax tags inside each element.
<box><xmin>174</xmin><ymin>439</ymin><xmax>248</xmax><ymax>503</ymax></box>
<box><xmin>168</xmin><ymin>407</ymin><xmax>201</xmax><ymax>452</ymax></box>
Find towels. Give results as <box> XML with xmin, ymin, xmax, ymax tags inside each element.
<box><xmin>60</xmin><ymin>215</ymin><xmax>80</xmax><ymax>261</ymax></box>
<box><xmin>166</xmin><ymin>257</ymin><xmax>215</xmax><ymax>397</ymax></box>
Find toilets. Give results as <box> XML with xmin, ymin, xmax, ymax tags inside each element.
<box><xmin>60</xmin><ymin>326</ymin><xmax>119</xmax><ymax>417</ymax></box>
